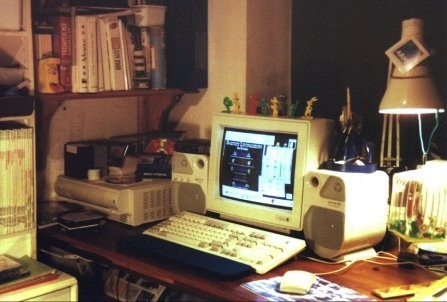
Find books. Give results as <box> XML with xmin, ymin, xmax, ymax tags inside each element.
<box><xmin>36</xmin><ymin>6</ymin><xmax>151</xmax><ymax>92</ymax></box>
<box><xmin>0</xmin><ymin>129</ymin><xmax>35</xmax><ymax>234</ymax></box>
<box><xmin>0</xmin><ymin>252</ymin><xmax>61</xmax><ymax>293</ymax></box>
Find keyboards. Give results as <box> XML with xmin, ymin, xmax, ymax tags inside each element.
<box><xmin>141</xmin><ymin>210</ymin><xmax>307</xmax><ymax>275</ymax></box>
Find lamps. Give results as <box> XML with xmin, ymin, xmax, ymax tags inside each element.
<box><xmin>378</xmin><ymin>17</ymin><xmax>445</xmax><ymax>168</ymax></box>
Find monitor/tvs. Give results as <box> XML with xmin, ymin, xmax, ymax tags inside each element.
<box><xmin>207</xmin><ymin>112</ymin><xmax>335</xmax><ymax>232</ymax></box>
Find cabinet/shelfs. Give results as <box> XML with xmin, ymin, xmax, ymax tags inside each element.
<box><xmin>0</xmin><ymin>1</ymin><xmax>185</xmax><ymax>301</ymax></box>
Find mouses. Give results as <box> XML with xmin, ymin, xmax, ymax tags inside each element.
<box><xmin>279</xmin><ymin>270</ymin><xmax>315</xmax><ymax>295</ymax></box>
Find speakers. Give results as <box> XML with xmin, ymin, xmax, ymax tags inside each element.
<box><xmin>170</xmin><ymin>152</ymin><xmax>208</xmax><ymax>215</ymax></box>
<box><xmin>302</xmin><ymin>169</ymin><xmax>389</xmax><ymax>267</ymax></box>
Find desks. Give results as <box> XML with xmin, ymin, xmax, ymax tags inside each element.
<box><xmin>45</xmin><ymin>198</ymin><xmax>447</xmax><ymax>302</ymax></box>
